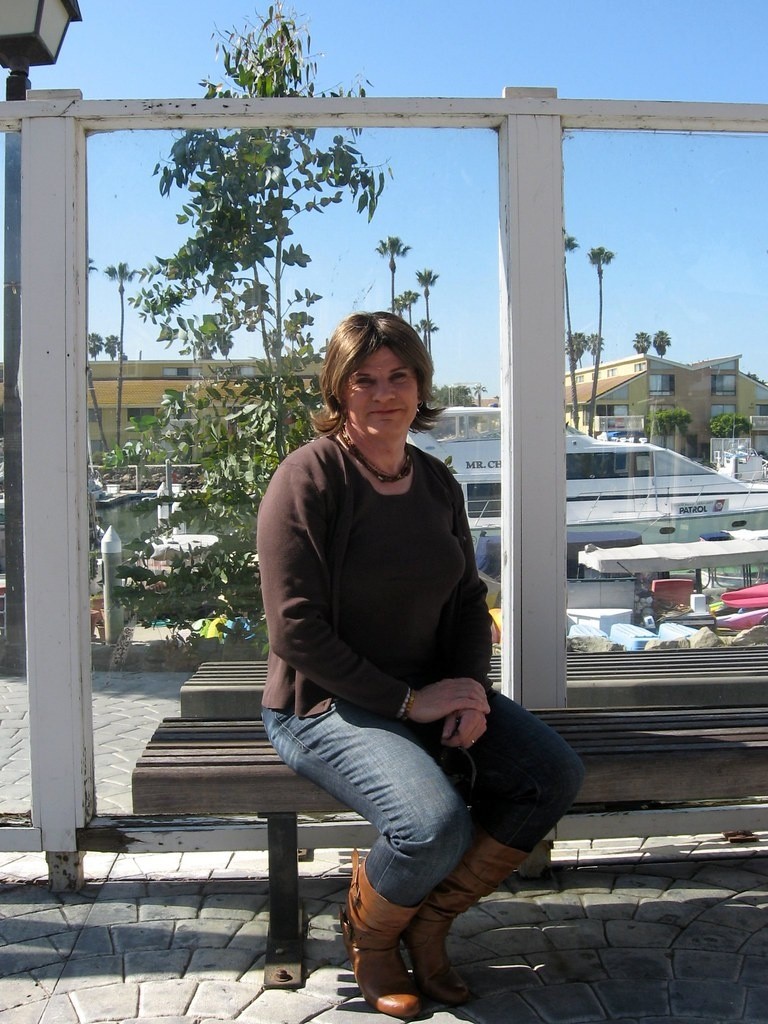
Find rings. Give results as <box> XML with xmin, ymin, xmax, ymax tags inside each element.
<box><xmin>472</xmin><ymin>739</ymin><xmax>475</xmax><ymax>743</ymax></box>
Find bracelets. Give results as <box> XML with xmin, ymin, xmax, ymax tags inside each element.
<box><xmin>397</xmin><ymin>688</ymin><xmax>415</xmax><ymax>720</ymax></box>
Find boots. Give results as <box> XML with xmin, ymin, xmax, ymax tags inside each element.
<box><xmin>400</xmin><ymin>832</ymin><xmax>530</xmax><ymax>1008</ymax></box>
<box><xmin>338</xmin><ymin>844</ymin><xmax>421</xmax><ymax>1019</ymax></box>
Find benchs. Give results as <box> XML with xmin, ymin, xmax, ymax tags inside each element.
<box><xmin>180</xmin><ymin>645</ymin><xmax>768</xmax><ymax>719</ymax></box>
<box><xmin>132</xmin><ymin>703</ymin><xmax>768</xmax><ymax>987</ymax></box>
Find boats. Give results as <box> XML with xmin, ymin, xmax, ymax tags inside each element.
<box><xmin>406</xmin><ymin>406</ymin><xmax>768</xmax><ymax>636</ymax></box>
<box><xmin>125</xmin><ymin>459</ymin><xmax>220</xmax><ymax>577</ymax></box>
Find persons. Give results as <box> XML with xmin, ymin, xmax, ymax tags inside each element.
<box><xmin>256</xmin><ymin>310</ymin><xmax>587</xmax><ymax>1017</ymax></box>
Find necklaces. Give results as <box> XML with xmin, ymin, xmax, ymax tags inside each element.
<box><xmin>342</xmin><ymin>430</ymin><xmax>412</xmax><ymax>482</ymax></box>
